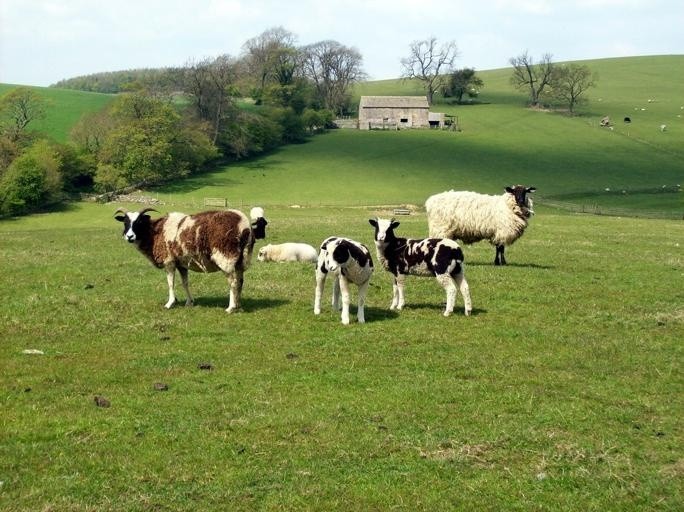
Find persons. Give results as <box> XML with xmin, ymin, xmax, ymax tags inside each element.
<box><xmin>600</xmin><ymin>115</ymin><xmax>609</xmax><ymax>126</ymax></box>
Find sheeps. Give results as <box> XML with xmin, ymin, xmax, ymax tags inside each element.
<box><xmin>624</xmin><ymin>117</ymin><xmax>631</xmax><ymax>123</ymax></box>
<box><xmin>111</xmin><ymin>205</ymin><xmax>254</xmax><ymax>314</ymax></box>
<box><xmin>368</xmin><ymin>214</ymin><xmax>474</xmax><ymax>318</ymax></box>
<box><xmin>660</xmin><ymin>124</ymin><xmax>666</xmax><ymax>133</ymax></box>
<box><xmin>423</xmin><ymin>184</ymin><xmax>537</xmax><ymax>265</ymax></box>
<box><xmin>257</xmin><ymin>241</ymin><xmax>319</xmax><ymax>265</ymax></box>
<box><xmin>312</xmin><ymin>235</ymin><xmax>376</xmax><ymax>324</ymax></box>
<box><xmin>250</xmin><ymin>206</ymin><xmax>268</xmax><ymax>238</ymax></box>
